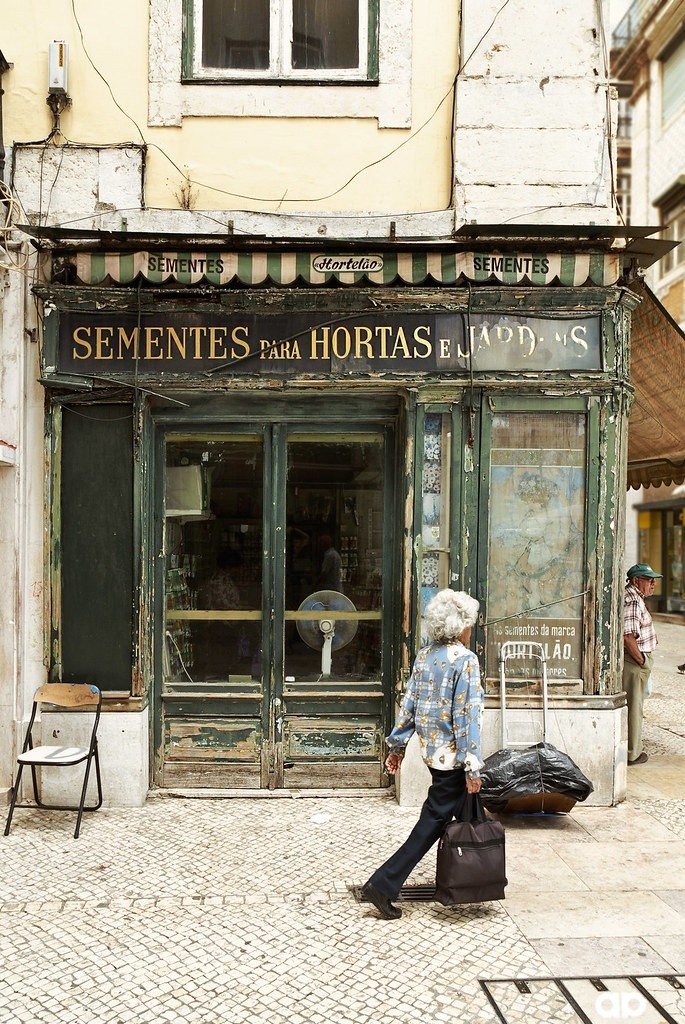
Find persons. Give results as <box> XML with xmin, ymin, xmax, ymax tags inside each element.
<box><xmin>361</xmin><ymin>588</ymin><xmax>509</xmax><ymax>919</ymax></box>
<box><xmin>625</xmin><ymin>563</ymin><xmax>664</xmax><ymax>766</ymax></box>
<box><xmin>316</xmin><ymin>535</ymin><xmax>345</xmax><ymax>592</ymax></box>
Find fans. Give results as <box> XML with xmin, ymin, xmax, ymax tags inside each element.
<box><xmin>295</xmin><ymin>589</ymin><xmax>359</xmax><ymax>675</ymax></box>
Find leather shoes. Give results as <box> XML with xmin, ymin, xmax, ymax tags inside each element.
<box><xmin>362</xmin><ymin>881</ymin><xmax>402</xmax><ymax>920</ymax></box>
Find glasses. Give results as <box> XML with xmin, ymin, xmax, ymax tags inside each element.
<box><xmin>637</xmin><ymin>576</ymin><xmax>657</xmax><ymax>581</ymax></box>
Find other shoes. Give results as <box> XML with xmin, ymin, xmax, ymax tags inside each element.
<box><xmin>629</xmin><ymin>752</ymin><xmax>648</xmax><ymax>764</ymax></box>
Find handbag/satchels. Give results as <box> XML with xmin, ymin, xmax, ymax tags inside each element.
<box><xmin>433</xmin><ymin>786</ymin><xmax>506</xmax><ymax>905</ymax></box>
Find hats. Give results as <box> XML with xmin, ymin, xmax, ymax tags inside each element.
<box><xmin>627</xmin><ymin>563</ymin><xmax>663</xmax><ymax>579</ymax></box>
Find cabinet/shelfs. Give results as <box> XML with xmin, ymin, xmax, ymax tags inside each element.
<box><xmin>348</xmin><ymin>587</ymin><xmax>382</xmax><ymax>676</ymax></box>
<box><xmin>340</xmin><ymin>540</ymin><xmax>359</xmax><ymax>583</ymax></box>
<box><xmin>214</xmin><ymin>516</ymin><xmax>320</xmax><ymax>584</ymax></box>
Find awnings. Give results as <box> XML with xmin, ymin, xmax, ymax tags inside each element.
<box><xmin>627</xmin><ymin>277</ymin><xmax>685</xmax><ymax>490</ymax></box>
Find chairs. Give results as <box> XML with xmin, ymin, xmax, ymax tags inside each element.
<box><xmin>3</xmin><ymin>683</ymin><xmax>102</xmax><ymax>839</ymax></box>
